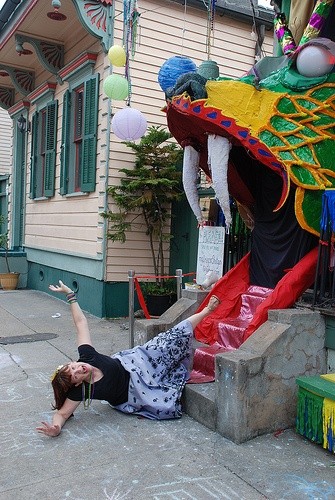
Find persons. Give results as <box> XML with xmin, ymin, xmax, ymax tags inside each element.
<box><xmin>35</xmin><ymin>280</ymin><xmax>222</xmax><ymax>437</ymax></box>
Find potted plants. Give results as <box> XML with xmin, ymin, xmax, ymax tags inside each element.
<box><xmin>99</xmin><ymin>124</ymin><xmax>183</xmax><ymax>318</ymax></box>
<box><xmin>0</xmin><ymin>215</ymin><xmax>21</xmax><ymax>291</ymax></box>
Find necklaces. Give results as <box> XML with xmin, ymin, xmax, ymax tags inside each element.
<box><xmin>81</xmin><ymin>368</ymin><xmax>94</xmax><ymax>410</ymax></box>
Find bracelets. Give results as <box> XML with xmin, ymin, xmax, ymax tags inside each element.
<box><xmin>65</xmin><ymin>291</ymin><xmax>78</xmax><ymax>304</ymax></box>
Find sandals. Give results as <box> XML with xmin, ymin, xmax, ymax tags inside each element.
<box><xmin>206</xmin><ymin>295</ymin><xmax>221</xmax><ymax>312</ymax></box>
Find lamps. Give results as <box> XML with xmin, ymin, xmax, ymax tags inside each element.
<box><xmin>17</xmin><ymin>112</ymin><xmax>30</xmax><ymax>135</ymax></box>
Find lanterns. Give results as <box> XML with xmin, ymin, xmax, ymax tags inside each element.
<box><xmin>103</xmin><ymin>75</ymin><xmax>128</xmax><ymax>101</ymax></box>
<box><xmin>108</xmin><ymin>45</ymin><xmax>126</xmax><ymax>67</ymax></box>
<box><xmin>112</xmin><ymin>107</ymin><xmax>147</xmax><ymax>141</ymax></box>
